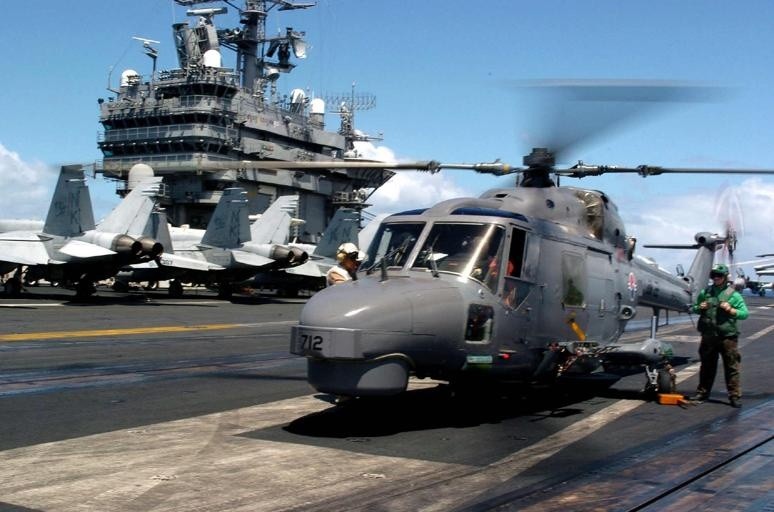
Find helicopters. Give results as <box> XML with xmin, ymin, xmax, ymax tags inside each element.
<box><xmin>206</xmin><ymin>78</ymin><xmax>745</xmax><ymax>429</ymax></box>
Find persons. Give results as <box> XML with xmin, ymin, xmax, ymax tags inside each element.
<box><xmin>451</xmin><ymin>233</ymin><xmax>514</xmax><ymax>306</ymax></box>
<box><xmin>688</xmin><ymin>264</ymin><xmax>750</xmax><ymax>407</ymax></box>
<box><xmin>325</xmin><ymin>242</ymin><xmax>359</xmax><ymax>288</ymax></box>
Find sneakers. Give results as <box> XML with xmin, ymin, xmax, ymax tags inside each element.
<box><xmin>684</xmin><ymin>393</ymin><xmax>708</xmax><ymax>400</ymax></box>
<box><xmin>732</xmin><ymin>398</ymin><xmax>742</xmax><ymax>407</ymax></box>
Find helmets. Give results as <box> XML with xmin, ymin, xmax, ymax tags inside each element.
<box><xmin>710</xmin><ymin>264</ymin><xmax>728</xmax><ymax>278</ymax></box>
<box><xmin>337</xmin><ymin>243</ymin><xmax>358</xmax><ymax>262</ymax></box>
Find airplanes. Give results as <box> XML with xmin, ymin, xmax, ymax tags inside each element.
<box><xmin>735</xmin><ymin>253</ymin><xmax>774</xmax><ymax>297</ymax></box>
<box><xmin>0</xmin><ymin>165</ymin><xmax>359</xmax><ymax>301</ymax></box>
